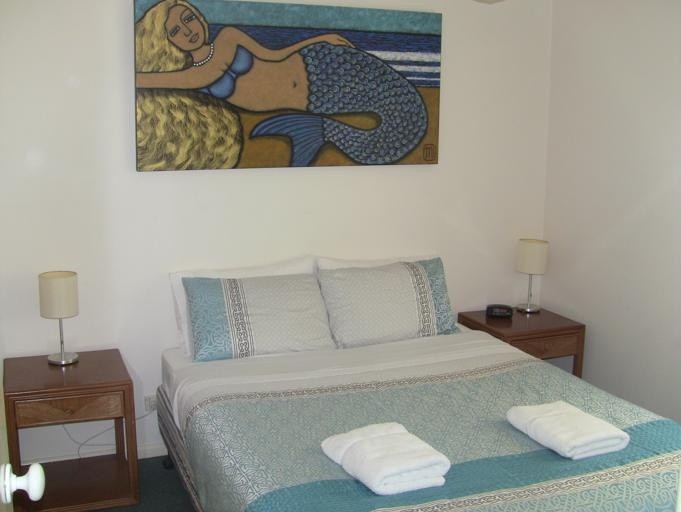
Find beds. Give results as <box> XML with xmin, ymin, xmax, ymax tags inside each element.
<box><xmin>157</xmin><ymin>345</ymin><xmax>681</xmax><ymax>512</ymax></box>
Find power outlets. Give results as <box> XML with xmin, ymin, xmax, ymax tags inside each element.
<box><xmin>145</xmin><ymin>396</ymin><xmax>159</xmax><ymax>410</ymax></box>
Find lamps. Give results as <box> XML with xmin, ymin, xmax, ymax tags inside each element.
<box><xmin>516</xmin><ymin>238</ymin><xmax>552</xmax><ymax>315</ymax></box>
<box><xmin>38</xmin><ymin>270</ymin><xmax>80</xmax><ymax>366</ymax></box>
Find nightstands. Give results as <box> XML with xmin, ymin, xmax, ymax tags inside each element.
<box><xmin>3</xmin><ymin>348</ymin><xmax>140</xmax><ymax>512</ymax></box>
<box><xmin>458</xmin><ymin>306</ymin><xmax>585</xmax><ymax>378</ymax></box>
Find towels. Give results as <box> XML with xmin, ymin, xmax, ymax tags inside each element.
<box><xmin>505</xmin><ymin>401</ymin><xmax>630</xmax><ymax>460</ymax></box>
<box><xmin>321</xmin><ymin>422</ymin><xmax>451</xmax><ymax>495</ymax></box>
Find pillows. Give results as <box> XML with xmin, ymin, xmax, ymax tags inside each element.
<box><xmin>317</xmin><ymin>256</ymin><xmax>459</xmax><ymax>350</ymax></box>
<box><xmin>181</xmin><ymin>276</ymin><xmax>339</xmax><ymax>362</ymax></box>
<box><xmin>170</xmin><ymin>256</ymin><xmax>316</xmax><ymax>360</ymax></box>
<box><xmin>316</xmin><ymin>254</ymin><xmax>435</xmax><ymax>269</ymax></box>
